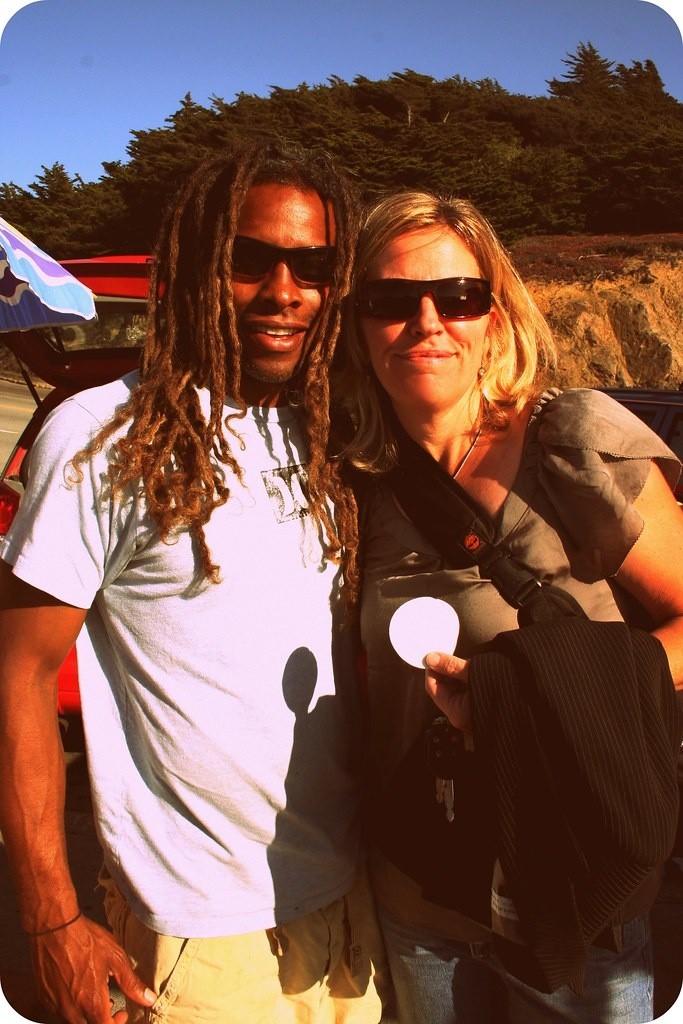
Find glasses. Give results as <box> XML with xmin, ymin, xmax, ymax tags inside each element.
<box><xmin>355</xmin><ymin>275</ymin><xmax>493</xmax><ymax>321</ymax></box>
<box><xmin>225</xmin><ymin>235</ymin><xmax>338</xmax><ymax>285</ymax></box>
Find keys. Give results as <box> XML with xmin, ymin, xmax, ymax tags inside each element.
<box><xmin>430</xmin><ymin>747</ymin><xmax>458</xmax><ymax>822</ymax></box>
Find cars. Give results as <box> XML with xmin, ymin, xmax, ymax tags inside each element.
<box><xmin>589</xmin><ymin>386</ymin><xmax>683</xmax><ymax>505</ymax></box>
<box><xmin>0</xmin><ymin>251</ymin><xmax>180</xmax><ymax>717</ymax></box>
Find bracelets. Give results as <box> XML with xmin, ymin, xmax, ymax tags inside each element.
<box><xmin>23</xmin><ymin>910</ymin><xmax>82</xmax><ymax>937</ymax></box>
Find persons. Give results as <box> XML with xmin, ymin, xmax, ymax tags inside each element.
<box><xmin>331</xmin><ymin>192</ymin><xmax>683</xmax><ymax>1024</ymax></box>
<box><xmin>0</xmin><ymin>138</ymin><xmax>390</xmax><ymax>1024</ymax></box>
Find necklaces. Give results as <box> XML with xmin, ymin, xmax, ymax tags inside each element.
<box><xmin>452</xmin><ymin>419</ymin><xmax>487</xmax><ymax>480</ymax></box>
